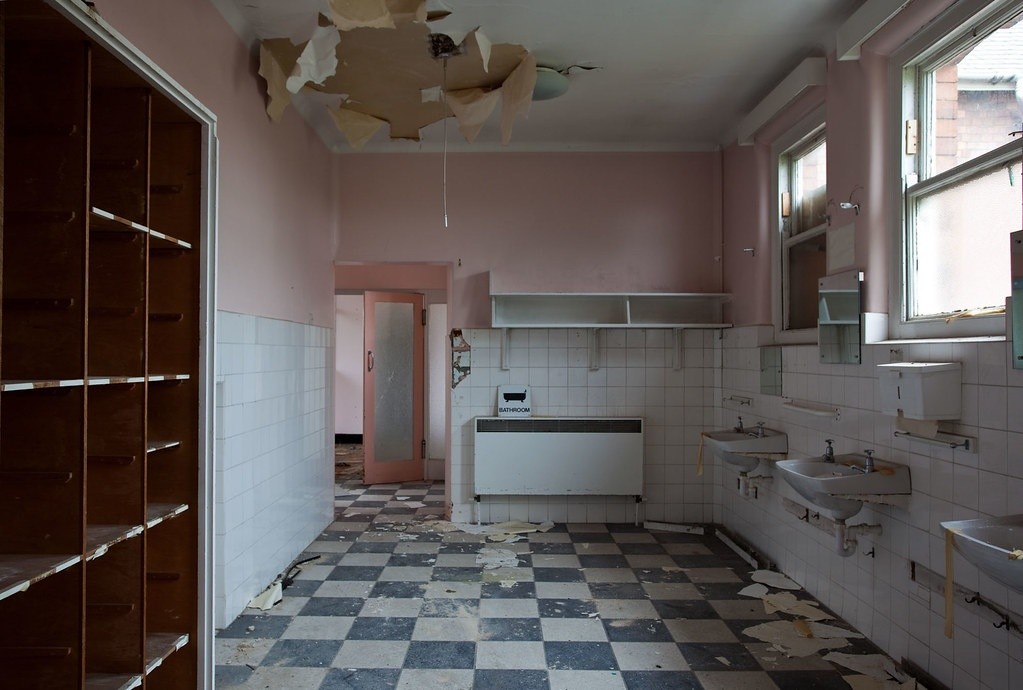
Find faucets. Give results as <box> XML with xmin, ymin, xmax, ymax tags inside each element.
<box><xmin>746</xmin><ymin>421</ymin><xmax>766</xmax><ymax>438</ymax></box>
<box><xmin>847</xmin><ymin>447</ymin><xmax>875</xmax><ymax>477</ymax></box>
<box><xmin>819</xmin><ymin>438</ymin><xmax>836</xmax><ymax>464</ymax></box>
<box><xmin>731</xmin><ymin>417</ymin><xmax>744</xmax><ymax>434</ymax></box>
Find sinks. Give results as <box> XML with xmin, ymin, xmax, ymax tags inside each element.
<box><xmin>701</xmin><ymin>425</ymin><xmax>790</xmax><ymax>470</ymax></box>
<box><xmin>773</xmin><ymin>450</ymin><xmax>912</xmax><ymax>510</ymax></box>
<box><xmin>940</xmin><ymin>509</ymin><xmax>1023</xmax><ymax>602</ymax></box>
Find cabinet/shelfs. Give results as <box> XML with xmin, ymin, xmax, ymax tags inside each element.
<box><xmin>487</xmin><ymin>269</ymin><xmax>735</xmax><ymax>372</ymax></box>
<box><xmin>0</xmin><ymin>0</ymin><xmax>219</xmax><ymax>690</ymax></box>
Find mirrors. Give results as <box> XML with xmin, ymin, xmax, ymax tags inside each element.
<box><xmin>817</xmin><ymin>267</ymin><xmax>862</xmax><ymax>364</ymax></box>
<box><xmin>759</xmin><ymin>346</ymin><xmax>783</xmax><ymax>395</ymax></box>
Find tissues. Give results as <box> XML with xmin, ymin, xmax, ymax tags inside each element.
<box><xmin>875</xmin><ymin>361</ymin><xmax>964</xmax><ymax>438</ymax></box>
